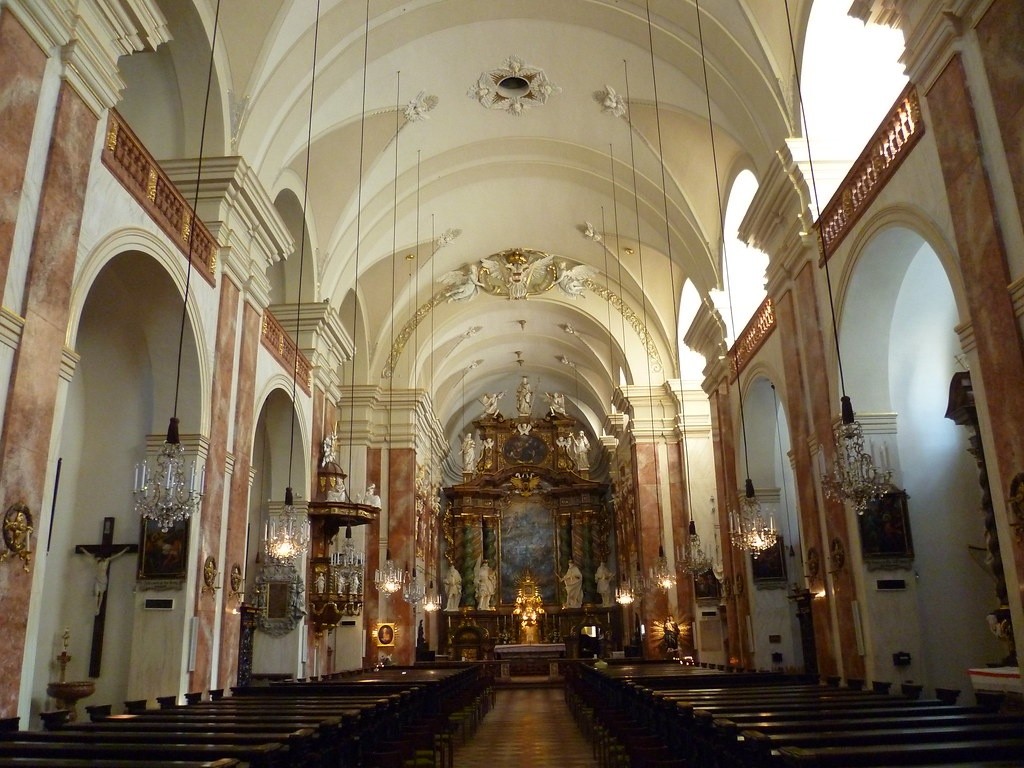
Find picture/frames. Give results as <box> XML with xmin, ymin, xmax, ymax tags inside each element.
<box><xmin>856</xmin><ymin>484</ymin><xmax>914</xmax><ymax>573</ymax></box>
<box><xmin>751</xmin><ymin>534</ymin><xmax>788</xmax><ymax>590</ymax></box>
<box><xmin>136</xmin><ymin>513</ymin><xmax>189</xmax><ymax>591</ymax></box>
<box><xmin>692</xmin><ymin>567</ymin><xmax>723</xmax><ymax>607</ymax></box>
<box><xmin>252</xmin><ymin>561</ymin><xmax>298</xmax><ymax>632</ymax></box>
<box><xmin>373</xmin><ymin>620</ymin><xmax>399</xmax><ymax>649</ymax></box>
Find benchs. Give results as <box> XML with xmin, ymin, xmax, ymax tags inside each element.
<box><xmin>0</xmin><ymin>662</ymin><xmax>495</xmax><ymax>768</ymax></box>
<box><xmin>567</xmin><ymin>658</ymin><xmax>1024</xmax><ymax>768</ymax></box>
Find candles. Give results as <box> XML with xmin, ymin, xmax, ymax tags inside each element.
<box><xmin>559</xmin><ymin>617</ymin><xmax>561</xmax><ymax>624</ymax></box>
<box><xmin>504</xmin><ymin>616</ymin><xmax>506</xmax><ymax>624</ymax></box>
<box><xmin>511</xmin><ymin>614</ymin><xmax>513</xmax><ymax>624</ymax></box>
<box><xmin>497</xmin><ymin>617</ymin><xmax>498</xmax><ymax>625</ymax></box>
<box><xmin>553</xmin><ymin>615</ymin><xmax>555</xmax><ymax>623</ymax></box>
<box><xmin>545</xmin><ymin>612</ymin><xmax>547</xmax><ymax>621</ymax></box>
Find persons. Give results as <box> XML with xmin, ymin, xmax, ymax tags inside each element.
<box><xmin>480</xmin><ymin>391</ymin><xmax>505</xmax><ymax>419</ymax></box>
<box><xmin>443</xmin><ymin>566</ymin><xmax>462</xmax><ymax>610</ymax></box>
<box><xmin>544</xmin><ymin>391</ymin><xmax>569</xmax><ymax>416</ymax></box>
<box><xmin>327</xmin><ymin>477</ymin><xmax>345</xmax><ymax>502</ymax></box>
<box><xmin>566</xmin><ymin>430</ymin><xmax>591</xmax><ymax>470</ymax></box>
<box><xmin>337</xmin><ymin>576</ymin><xmax>345</xmax><ymax>593</ymax></box>
<box><xmin>515</xmin><ymin>377</ymin><xmax>532</xmax><ymax>416</ymax></box>
<box><xmin>664</xmin><ymin>615</ymin><xmax>680</xmax><ymax>648</ymax></box>
<box><xmin>77</xmin><ymin>547</ymin><xmax>129</xmax><ymax>616</ymax></box>
<box><xmin>314</xmin><ymin>573</ymin><xmax>324</xmax><ymax>594</ymax></box>
<box><xmin>595</xmin><ymin>561</ymin><xmax>617</xmax><ymax>607</ymax></box>
<box><xmin>364</xmin><ymin>483</ymin><xmax>381</xmax><ymax>507</ymax></box>
<box><xmin>473</xmin><ymin>553</ymin><xmax>497</xmax><ymax>610</ymax></box>
<box><xmin>349</xmin><ymin>575</ymin><xmax>360</xmax><ymax>594</ymax></box>
<box><xmin>458</xmin><ymin>432</ymin><xmax>475</xmax><ymax>471</ymax></box>
<box><xmin>554</xmin><ymin>560</ymin><xmax>584</xmax><ymax>608</ymax></box>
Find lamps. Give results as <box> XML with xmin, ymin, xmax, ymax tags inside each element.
<box><xmin>260</xmin><ymin>0</ymin><xmax>321</xmax><ymax>559</ymax></box>
<box><xmin>601</xmin><ymin>0</ymin><xmax>779</xmax><ymax>609</ymax></box>
<box><xmin>328</xmin><ymin>0</ymin><xmax>369</xmax><ymax>572</ymax></box>
<box><xmin>783</xmin><ymin>0</ymin><xmax>892</xmax><ymax>516</ymax></box>
<box><xmin>373</xmin><ymin>72</ymin><xmax>442</xmax><ymax>614</ymax></box>
<box><xmin>132</xmin><ymin>0</ymin><xmax>222</xmax><ymax>529</ymax></box>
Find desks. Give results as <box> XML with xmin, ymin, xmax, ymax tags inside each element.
<box><xmin>494</xmin><ymin>644</ymin><xmax>567</xmax><ymax>679</ymax></box>
